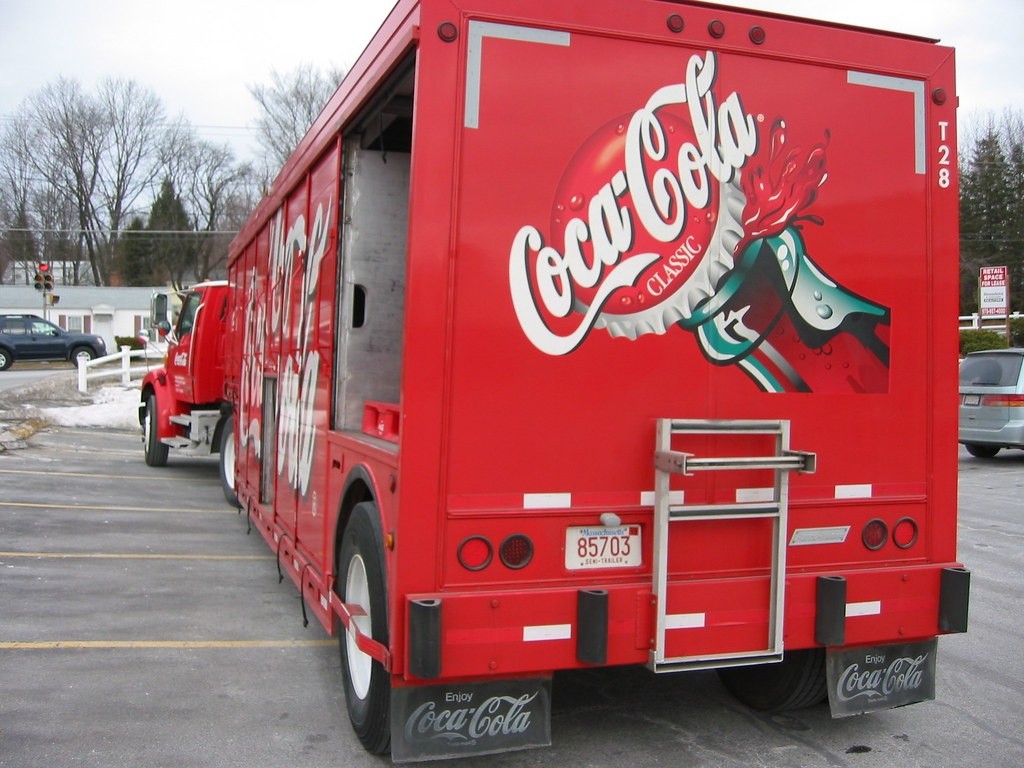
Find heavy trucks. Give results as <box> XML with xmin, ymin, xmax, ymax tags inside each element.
<box><xmin>137</xmin><ymin>0</ymin><xmax>970</xmax><ymax>768</ymax></box>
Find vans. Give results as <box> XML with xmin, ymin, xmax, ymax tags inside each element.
<box><xmin>958</xmin><ymin>349</ymin><xmax>1024</xmax><ymax>458</ymax></box>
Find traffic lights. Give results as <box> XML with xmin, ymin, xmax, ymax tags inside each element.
<box><xmin>49</xmin><ymin>294</ymin><xmax>59</xmax><ymax>304</ymax></box>
<box><xmin>35</xmin><ymin>264</ymin><xmax>54</xmax><ymax>290</ymax></box>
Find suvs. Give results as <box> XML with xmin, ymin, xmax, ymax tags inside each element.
<box><xmin>0</xmin><ymin>314</ymin><xmax>108</xmax><ymax>370</ymax></box>
